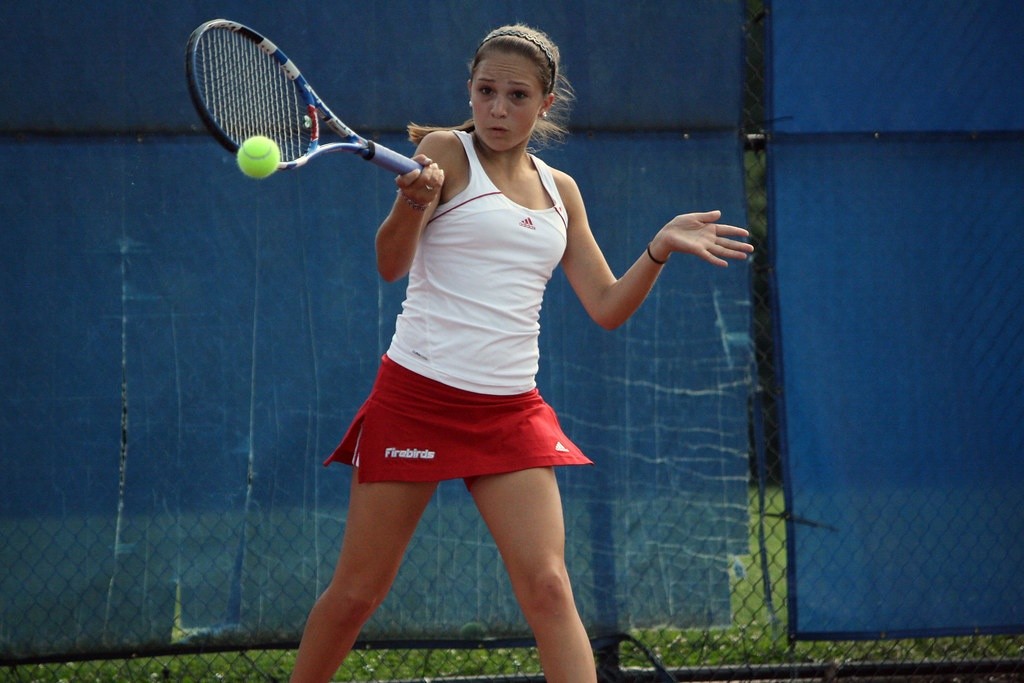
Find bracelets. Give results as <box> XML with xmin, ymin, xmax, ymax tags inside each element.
<box><xmin>398</xmin><ymin>195</ymin><xmax>431</xmax><ymax>209</ymax></box>
<box><xmin>648</xmin><ymin>244</ymin><xmax>671</xmax><ymax>269</ymax></box>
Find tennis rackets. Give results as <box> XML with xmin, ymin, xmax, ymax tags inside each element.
<box><xmin>183</xmin><ymin>17</ymin><xmax>423</xmax><ymax>172</ymax></box>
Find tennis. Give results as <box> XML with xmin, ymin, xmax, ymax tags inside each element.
<box><xmin>235</xmin><ymin>135</ymin><xmax>282</xmax><ymax>181</ymax></box>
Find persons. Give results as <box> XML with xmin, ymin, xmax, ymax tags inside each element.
<box><xmin>291</xmin><ymin>24</ymin><xmax>752</xmax><ymax>683</ymax></box>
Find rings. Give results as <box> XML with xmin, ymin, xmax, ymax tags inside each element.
<box><xmin>425</xmin><ymin>183</ymin><xmax>432</xmax><ymax>190</ymax></box>
